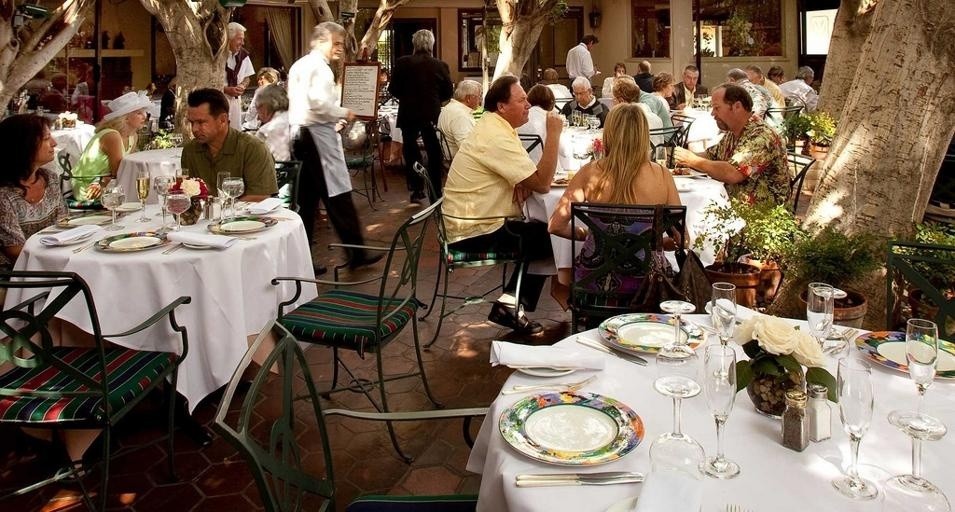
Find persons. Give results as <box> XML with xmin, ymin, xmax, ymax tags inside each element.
<box><xmin>729</xmin><ymin>64</ymin><xmax>818</xmax><ymax>157</ymax></box>
<box><xmin>438</xmin><ymin>79</ymin><xmax>483</xmax><ymax>162</ymax></box>
<box><xmin>388</xmin><ymin>28</ymin><xmax>453</xmax><ymax>205</ymax></box>
<box><xmin>673</xmin><ymin>83</ymin><xmax>789</xmax><ymax>263</ymax></box>
<box><xmin>602</xmin><ymin>61</ymin><xmax>708</xmax><ymax>161</ymax></box>
<box><xmin>565</xmin><ymin>34</ymin><xmax>598</xmax><ymax>91</ymax></box>
<box><xmin>287</xmin><ymin>22</ymin><xmax>383</xmax><ymax>274</ymax></box>
<box><xmin>224</xmin><ymin>22</ymin><xmax>291</xmax><ymax>200</ymax></box>
<box><xmin>159</xmin><ymin>78</ymin><xmax>178</xmax><ymax>129</ymax></box>
<box><xmin>181</xmin><ymin>88</ymin><xmax>279</xmax><ymax>203</ymax></box>
<box><xmin>442</xmin><ymin>77</ymin><xmax>564</xmax><ymax>335</ymax></box>
<box><xmin>515</xmin><ymin>67</ymin><xmax>611</xmax><ymax>166</ymax></box>
<box><xmin>26</xmin><ymin>65</ymin><xmax>95</xmax><ymax>114</ymax></box>
<box><xmin>73</xmin><ymin>92</ymin><xmax>152</xmax><ymax>206</ymax></box>
<box><xmin>1</xmin><ymin>114</ymin><xmax>67</xmax><ymax>279</ymax></box>
<box><xmin>548</xmin><ymin>104</ymin><xmax>690</xmax><ymax>307</ymax></box>
<box><xmin>334</xmin><ymin>73</ymin><xmax>366</xmax><ymax>146</ymax></box>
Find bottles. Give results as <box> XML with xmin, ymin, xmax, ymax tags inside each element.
<box><xmin>209</xmin><ymin>197</ymin><xmax>221</xmax><ymax>221</ymax></box>
<box><xmin>781</xmin><ymin>390</ymin><xmax>810</xmax><ymax>452</ymax></box>
<box><xmin>808</xmin><ymin>384</ymin><xmax>833</xmax><ymax>442</ymax></box>
<box><xmin>203</xmin><ymin>196</ymin><xmax>213</xmax><ymax>220</ymax></box>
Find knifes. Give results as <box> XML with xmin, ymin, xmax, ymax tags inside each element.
<box><xmin>516</xmin><ymin>471</ymin><xmax>643</xmax><ymax>480</ymax></box>
<box><xmin>576</xmin><ymin>336</ymin><xmax>648</xmax><ymax>367</ymax></box>
<box><xmin>515</xmin><ymin>478</ymin><xmax>644</xmax><ymax>487</ymax></box>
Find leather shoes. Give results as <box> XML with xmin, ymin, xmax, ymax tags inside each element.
<box><xmin>410</xmin><ymin>192</ymin><xmax>426</xmax><ymax>202</ymax></box>
<box><xmin>313</xmin><ymin>265</ymin><xmax>328</xmax><ymax>276</ymax></box>
<box><xmin>348</xmin><ymin>253</ymin><xmax>385</xmax><ymax>271</ymax></box>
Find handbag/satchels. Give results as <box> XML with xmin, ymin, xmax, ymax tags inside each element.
<box><xmin>629</xmin><ymin>249</ymin><xmax>714</xmax><ymax>313</ymax></box>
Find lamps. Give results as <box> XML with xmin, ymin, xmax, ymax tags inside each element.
<box><xmin>589</xmin><ymin>5</ymin><xmax>602</xmax><ymax>35</ymax></box>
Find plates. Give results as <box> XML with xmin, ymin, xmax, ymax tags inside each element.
<box><xmin>207</xmin><ymin>215</ymin><xmax>278</xmax><ymax>234</ymax></box>
<box><xmin>550</xmin><ymin>183</ymin><xmax>570</xmax><ymax>187</ymax></box>
<box><xmin>54</xmin><ymin>209</ymin><xmax>129</xmax><ymax>229</ymax></box>
<box><xmin>116</xmin><ymin>203</ymin><xmax>142</xmax><ymax>212</ymax></box>
<box><xmin>674</xmin><ymin>178</ymin><xmax>695</xmax><ymax>191</ymax></box>
<box><xmin>93</xmin><ymin>231</ymin><xmax>172</xmax><ymax>252</ymax></box>
<box><xmin>517</xmin><ymin>369</ymin><xmax>576</xmax><ymax>377</ymax></box>
<box><xmin>54</xmin><ymin>231</ymin><xmax>98</xmax><ymax>245</ymax></box>
<box><xmin>669</xmin><ymin>168</ymin><xmax>694</xmax><ymax>178</ymax></box>
<box><xmin>498</xmin><ymin>391</ymin><xmax>645</xmax><ymax>467</ymax></box>
<box><xmin>182</xmin><ymin>243</ymin><xmax>213</xmax><ymax>250</ymax></box>
<box><xmin>598</xmin><ymin>313</ymin><xmax>708</xmax><ymax>353</ymax></box>
<box><xmin>855</xmin><ymin>331</ymin><xmax>955</xmax><ymax>380</ymax></box>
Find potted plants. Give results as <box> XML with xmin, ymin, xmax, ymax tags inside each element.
<box><xmin>785</xmin><ymin>221</ymin><xmax>876</xmax><ymax>328</ymax></box>
<box><xmin>883</xmin><ymin>219</ymin><xmax>954</xmax><ymax>339</ymax></box>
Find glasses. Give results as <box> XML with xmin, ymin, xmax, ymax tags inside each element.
<box><xmin>572</xmin><ymin>89</ymin><xmax>590</xmax><ymax>95</ymax></box>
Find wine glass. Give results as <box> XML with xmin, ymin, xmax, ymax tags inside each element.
<box><xmin>572</xmin><ymin>110</ymin><xmax>601</xmax><ymax>137</ymax></box>
<box><xmin>175</xmin><ymin>168</ymin><xmax>190</xmax><ymax>179</ymax></box>
<box><xmin>831</xmin><ymin>357</ymin><xmax>878</xmax><ymax>501</ymax></box>
<box><xmin>656</xmin><ymin>300</ymin><xmax>699</xmax><ymax>381</ymax></box>
<box><xmin>905</xmin><ymin>318</ymin><xmax>938</xmax><ymax>413</ymax></box>
<box><xmin>165</xmin><ymin>190</ymin><xmax>191</xmax><ymax>232</ymax></box>
<box><xmin>805</xmin><ymin>282</ymin><xmax>835</xmax><ymax>350</ymax></box>
<box><xmin>216</xmin><ymin>171</ymin><xmax>231</xmax><ymax>209</ymax></box>
<box><xmin>698</xmin><ymin>344</ymin><xmax>740</xmax><ymax>480</ymax></box>
<box><xmin>559</xmin><ymin>114</ymin><xmax>569</xmax><ymax>133</ymax></box>
<box><xmin>134</xmin><ymin>172</ymin><xmax>152</xmax><ymax>223</ymax></box>
<box><xmin>881</xmin><ymin>409</ymin><xmax>951</xmax><ymax>512</ymax></box>
<box><xmin>101</xmin><ymin>184</ymin><xmax>126</xmax><ymax>232</ymax></box>
<box><xmin>710</xmin><ymin>282</ymin><xmax>737</xmax><ymax>379</ymax></box>
<box><xmin>221</xmin><ymin>177</ymin><xmax>245</xmax><ymax>218</ymax></box>
<box><xmin>693</xmin><ymin>94</ymin><xmax>711</xmax><ymax>112</ymax></box>
<box><xmin>649</xmin><ymin>375</ymin><xmax>707</xmax><ymax>483</ymax></box>
<box><xmin>169</xmin><ymin>133</ymin><xmax>183</xmax><ymax>158</ymax></box>
<box><xmin>153</xmin><ymin>176</ymin><xmax>175</xmax><ymax>233</ymax></box>
<box><xmin>808</xmin><ymin>287</ymin><xmax>850</xmax><ymax>364</ymax></box>
<box><xmin>656</xmin><ymin>147</ymin><xmax>667</xmax><ymax>168</ymax></box>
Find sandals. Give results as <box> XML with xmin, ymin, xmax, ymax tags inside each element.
<box><xmin>487</xmin><ymin>299</ymin><xmax>544</xmax><ymax>334</ymax></box>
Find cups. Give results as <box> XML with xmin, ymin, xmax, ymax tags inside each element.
<box><xmin>55</xmin><ymin>119</ymin><xmax>63</xmax><ymax>130</ymax></box>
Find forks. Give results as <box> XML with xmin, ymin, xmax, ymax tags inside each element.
<box><xmin>500</xmin><ymin>375</ymin><xmax>596</xmax><ymax>396</ymax></box>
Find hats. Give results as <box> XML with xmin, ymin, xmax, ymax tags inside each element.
<box><xmin>103</xmin><ymin>91</ymin><xmax>153</xmax><ymax>121</ymax></box>
